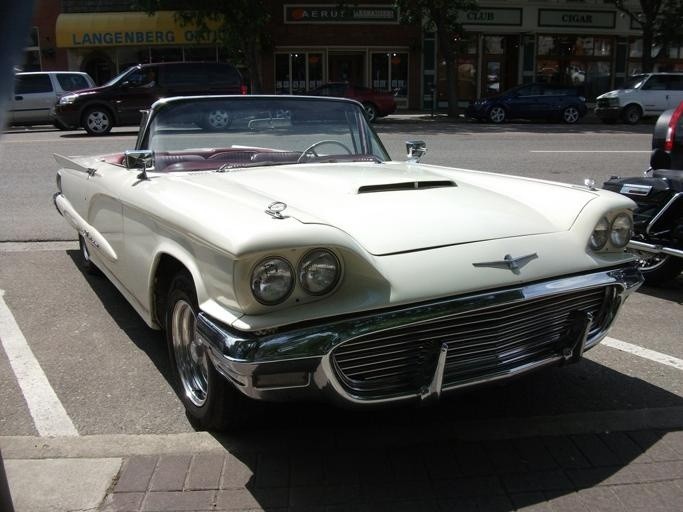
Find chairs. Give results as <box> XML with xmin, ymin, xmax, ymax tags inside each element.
<box><xmin>149</xmin><ymin>147</ymin><xmax>308</xmax><ymax>169</ymax></box>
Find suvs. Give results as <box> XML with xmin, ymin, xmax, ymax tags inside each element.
<box><xmin>48</xmin><ymin>60</ymin><xmax>251</xmax><ymax>133</ymax></box>
<box><xmin>595</xmin><ymin>73</ymin><xmax>682</xmax><ymax>125</ymax></box>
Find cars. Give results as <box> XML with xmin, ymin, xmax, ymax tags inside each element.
<box><xmin>467</xmin><ymin>84</ymin><xmax>590</xmax><ymax>122</ymax></box>
<box><xmin>291</xmin><ymin>83</ymin><xmax>395</xmax><ymax>125</ymax></box>
<box><xmin>10</xmin><ymin>70</ymin><xmax>95</xmax><ymax>126</ymax></box>
<box><xmin>52</xmin><ymin>94</ymin><xmax>643</xmax><ymax>430</ymax></box>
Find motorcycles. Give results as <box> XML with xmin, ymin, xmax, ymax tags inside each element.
<box><xmin>604</xmin><ymin>100</ymin><xmax>683</xmax><ymax>283</ymax></box>
